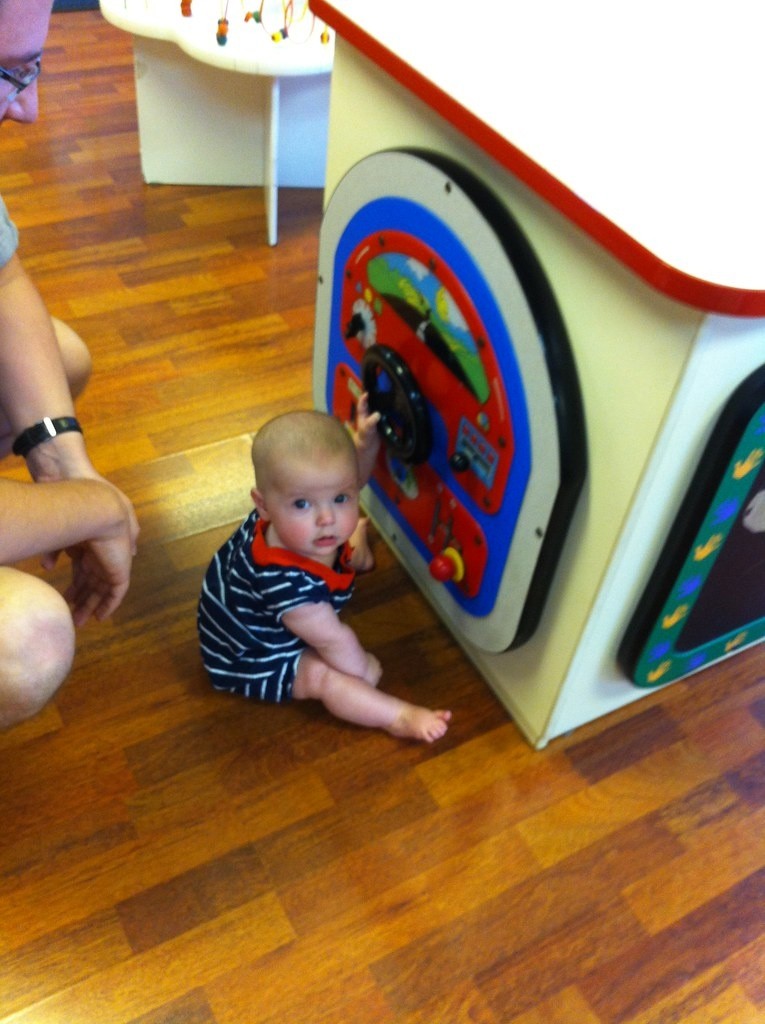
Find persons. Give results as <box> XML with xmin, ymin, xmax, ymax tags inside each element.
<box><xmin>0</xmin><ymin>0</ymin><xmax>140</xmax><ymax>732</ymax></box>
<box><xmin>195</xmin><ymin>392</ymin><xmax>451</xmax><ymax>744</ymax></box>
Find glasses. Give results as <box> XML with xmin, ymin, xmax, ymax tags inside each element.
<box><xmin>0</xmin><ymin>64</ymin><xmax>41</xmax><ymax>94</ymax></box>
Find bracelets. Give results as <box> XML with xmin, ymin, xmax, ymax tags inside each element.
<box><xmin>12</xmin><ymin>416</ymin><xmax>83</xmax><ymax>458</ymax></box>
